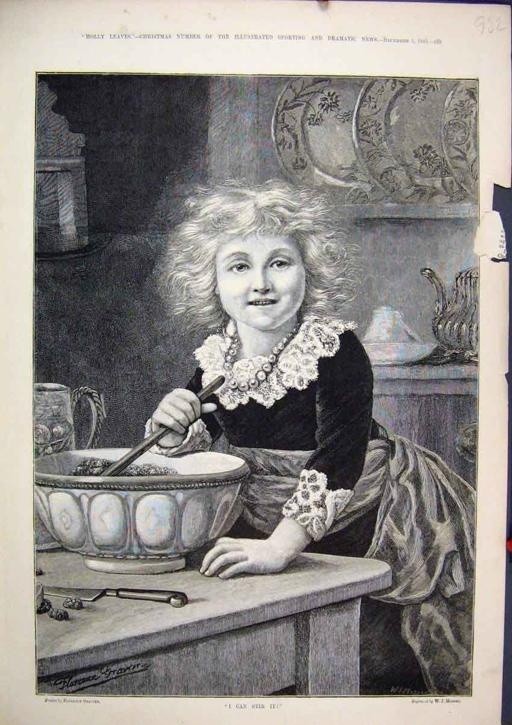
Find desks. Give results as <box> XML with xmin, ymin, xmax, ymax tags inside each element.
<box><xmin>34</xmin><ymin>539</ymin><xmax>395</xmax><ymax>697</ymax></box>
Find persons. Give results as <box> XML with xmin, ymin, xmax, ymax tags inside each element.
<box><xmin>145</xmin><ymin>178</ymin><xmax>472</xmax><ymax>697</ymax></box>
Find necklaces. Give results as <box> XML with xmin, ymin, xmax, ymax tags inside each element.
<box><xmin>223</xmin><ymin>325</ymin><xmax>300</xmax><ymax>393</ymax></box>
